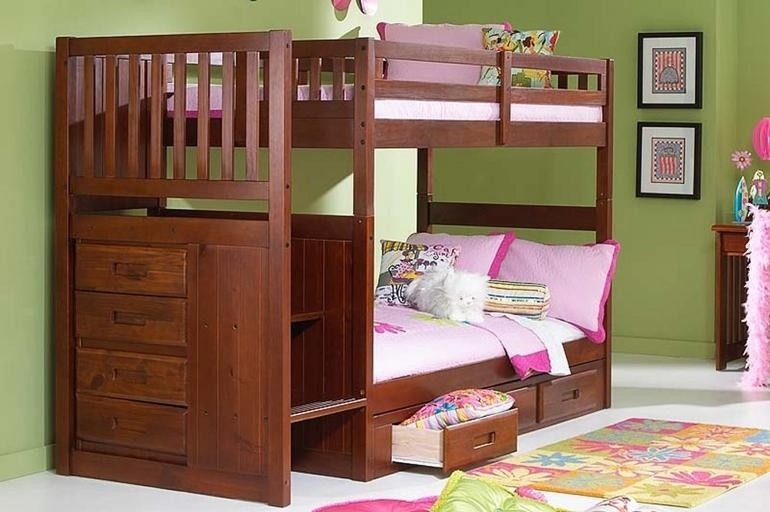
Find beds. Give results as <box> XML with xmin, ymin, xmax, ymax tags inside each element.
<box><xmin>48</xmin><ymin>32</ymin><xmax>614</xmax><ymax>503</ymax></box>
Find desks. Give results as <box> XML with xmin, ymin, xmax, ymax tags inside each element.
<box><xmin>710</xmin><ymin>224</ymin><xmax>748</xmax><ymax>370</ymax></box>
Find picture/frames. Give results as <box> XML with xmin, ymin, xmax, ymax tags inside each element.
<box><xmin>638</xmin><ymin>32</ymin><xmax>703</xmax><ymax>109</ymax></box>
<box><xmin>635</xmin><ymin>121</ymin><xmax>702</xmax><ymax>200</ymax></box>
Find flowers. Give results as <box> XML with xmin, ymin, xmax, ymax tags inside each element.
<box><xmin>733</xmin><ymin>149</ymin><xmax>750</xmax><ymax>172</ymax></box>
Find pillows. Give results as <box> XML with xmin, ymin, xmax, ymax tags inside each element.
<box><xmin>405</xmin><ymin>231</ymin><xmax>511</xmax><ymax>314</ymax></box>
<box><xmin>375</xmin><ymin>20</ymin><xmax>509</xmax><ymax>86</ymax></box>
<box><xmin>400</xmin><ymin>388</ymin><xmax>516</xmax><ymax>432</ymax></box>
<box><xmin>378</xmin><ymin>238</ymin><xmax>460</xmax><ymax>313</ymax></box>
<box><xmin>478</xmin><ymin>27</ymin><xmax>560</xmax><ymax>88</ymax></box>
<box><xmin>490</xmin><ymin>231</ymin><xmax>620</xmax><ymax>345</ymax></box>
<box><xmin>430</xmin><ymin>470</ymin><xmax>557</xmax><ymax>512</ymax></box>
<box><xmin>484</xmin><ymin>279</ymin><xmax>550</xmax><ymax>321</ymax></box>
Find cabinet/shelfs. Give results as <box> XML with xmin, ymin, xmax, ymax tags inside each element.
<box><xmin>72</xmin><ymin>235</ymin><xmax>266</xmax><ymax>476</ymax></box>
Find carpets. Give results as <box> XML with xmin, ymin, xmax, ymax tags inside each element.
<box><xmin>312</xmin><ymin>489</ymin><xmax>545</xmax><ymax>511</ymax></box>
<box><xmin>465</xmin><ymin>417</ymin><xmax>770</xmax><ymax>507</ymax></box>
